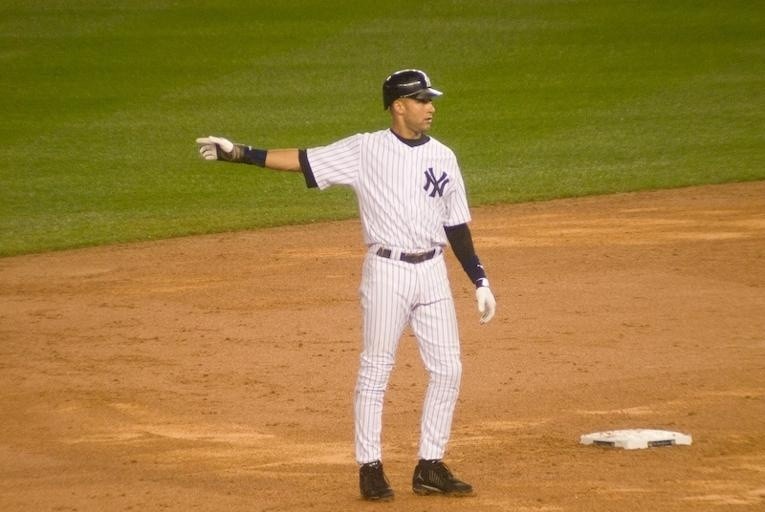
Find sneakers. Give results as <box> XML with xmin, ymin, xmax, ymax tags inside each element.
<box><xmin>359</xmin><ymin>460</ymin><xmax>394</xmax><ymax>501</ymax></box>
<box><xmin>412</xmin><ymin>460</ymin><xmax>473</xmax><ymax>496</ymax></box>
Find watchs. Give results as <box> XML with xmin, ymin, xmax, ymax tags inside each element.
<box><xmin>476</xmin><ymin>279</ymin><xmax>489</xmax><ymax>287</ymax></box>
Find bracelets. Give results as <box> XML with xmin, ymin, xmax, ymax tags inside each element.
<box><xmin>468</xmin><ymin>265</ymin><xmax>486</xmax><ymax>282</ymax></box>
<box><xmin>244</xmin><ymin>146</ymin><xmax>267</xmax><ymax>168</ymax></box>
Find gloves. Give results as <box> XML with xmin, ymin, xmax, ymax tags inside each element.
<box><xmin>196</xmin><ymin>135</ymin><xmax>234</xmax><ymax>160</ymax></box>
<box><xmin>475</xmin><ymin>287</ymin><xmax>496</xmax><ymax>325</ymax></box>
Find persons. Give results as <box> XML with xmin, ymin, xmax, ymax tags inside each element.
<box><xmin>195</xmin><ymin>70</ymin><xmax>495</xmax><ymax>501</ymax></box>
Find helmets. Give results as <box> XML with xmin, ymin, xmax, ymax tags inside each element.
<box><xmin>382</xmin><ymin>69</ymin><xmax>443</xmax><ymax>111</ymax></box>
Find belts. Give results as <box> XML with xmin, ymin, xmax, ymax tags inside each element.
<box><xmin>377</xmin><ymin>247</ymin><xmax>435</xmax><ymax>263</ymax></box>
